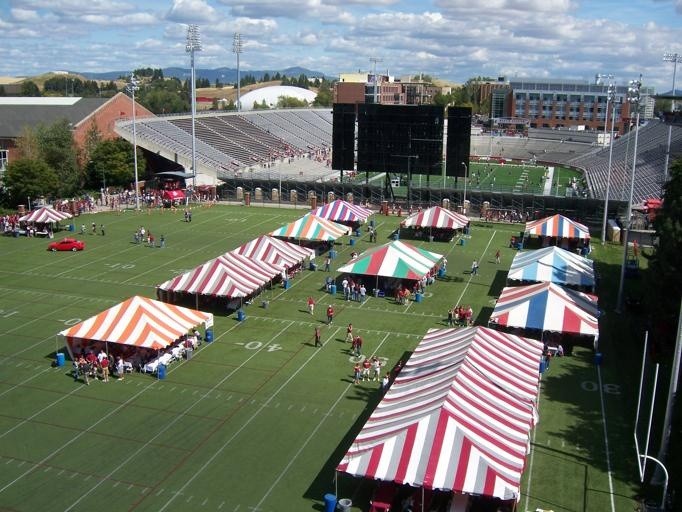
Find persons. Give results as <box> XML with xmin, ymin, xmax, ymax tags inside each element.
<box><xmin>387</xmin><ymin>371</ymin><xmax>390</xmax><ymax>379</ymax></box>
<box><xmin>510</xmin><ymin>236</ymin><xmax>515</xmax><ymax>248</ymax></box>
<box><xmin>382</xmin><ymin>376</ymin><xmax>389</xmax><ymax>394</ymax></box>
<box><xmin>150</xmin><ymin>237</ymin><xmax>155</xmax><ymax>245</ymax></box>
<box><xmin>71</xmin><ymin>349</ymin><xmax>125</xmax><ymax>386</ymax></box>
<box><xmin>314</xmin><ymin>327</ymin><xmax>322</xmax><ymax>347</ymax></box>
<box><xmin>361</xmin><ymin>358</ymin><xmax>372</xmax><ymax>382</ymax></box>
<box><xmin>350</xmin><ymin>336</ymin><xmax>358</xmax><ymax>354</ymax></box>
<box><xmin>0</xmin><ymin>179</ymin><xmax>192</xmax><ymax>239</ymax></box>
<box><xmin>323</xmin><ymin>257</ymin><xmax>330</xmax><ymax>272</ymax></box>
<box><xmin>327</xmin><ymin>305</ymin><xmax>333</xmax><ymax>327</ymax></box>
<box><xmin>353</xmin><ymin>363</ymin><xmax>361</xmax><ymax>385</ymax></box>
<box><xmin>393</xmin><ymin>256</ymin><xmax>448</xmax><ymax>305</ymax></box>
<box><xmin>146</xmin><ymin>232</ymin><xmax>149</xmax><ymax>242</ymax></box>
<box><xmin>193</xmin><ymin>329</ymin><xmax>202</xmax><ymax>345</ymax></box>
<box><xmin>356</xmin><ymin>335</ymin><xmax>362</xmax><ymax>357</ymax></box>
<box><xmin>370</xmin><ymin>229</ymin><xmax>374</xmax><ymax>242</ymax></box>
<box><xmin>471</xmin><ymin>259</ymin><xmax>479</xmax><ymax>275</ymax></box>
<box><xmin>140</xmin><ymin>225</ymin><xmax>145</xmax><ymax>240</ymax></box>
<box><xmin>372</xmin><ymin>230</ymin><xmax>377</xmax><ymax>242</ymax></box>
<box><xmin>495</xmin><ymin>250</ymin><xmax>500</xmax><ymax>264</ymax></box>
<box><xmin>342</xmin><ymin>277</ymin><xmax>366</xmax><ymax>304</ymax></box>
<box><xmin>372</xmin><ymin>358</ymin><xmax>381</xmax><ymax>381</ymax></box>
<box><xmin>307</xmin><ymin>296</ymin><xmax>315</xmax><ymax>315</ymax></box>
<box><xmin>447</xmin><ymin>304</ymin><xmax>472</xmax><ymax>326</ymax></box>
<box><xmin>345</xmin><ymin>323</ymin><xmax>353</xmax><ymax>343</ymax></box>
<box><xmin>134</xmin><ymin>231</ymin><xmax>140</xmax><ymax>244</ymax></box>
<box><xmin>160</xmin><ymin>234</ymin><xmax>166</xmax><ymax>248</ymax></box>
<box><xmin>545</xmin><ymin>350</ymin><xmax>551</xmax><ymax>370</ymax></box>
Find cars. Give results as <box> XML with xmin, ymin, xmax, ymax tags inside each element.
<box><xmin>48</xmin><ymin>238</ymin><xmax>85</xmax><ymax>252</ymax></box>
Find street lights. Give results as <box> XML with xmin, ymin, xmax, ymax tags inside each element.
<box><xmin>185</xmin><ymin>23</ymin><xmax>203</xmax><ymax>186</ymax></box>
<box><xmin>125</xmin><ymin>73</ymin><xmax>142</xmax><ymax>210</ymax></box>
<box><xmin>232</xmin><ymin>32</ymin><xmax>244</xmax><ymax>112</ymax></box>
<box><xmin>595</xmin><ymin>53</ymin><xmax>682</xmax><ymax>314</ymax></box>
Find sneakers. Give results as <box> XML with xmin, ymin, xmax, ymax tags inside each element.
<box><xmin>95</xmin><ymin>376</ymin><xmax>124</xmax><ymax>382</ymax></box>
<box><xmin>356</xmin><ymin>377</ymin><xmax>380</xmax><ymax>384</ymax></box>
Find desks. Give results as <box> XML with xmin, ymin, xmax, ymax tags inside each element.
<box><xmin>369</xmin><ymin>480</ymin><xmax>472</xmax><ymax>512</ymax></box>
<box><xmin>124</xmin><ymin>338</ymin><xmax>197</xmax><ymax>374</ymax></box>
